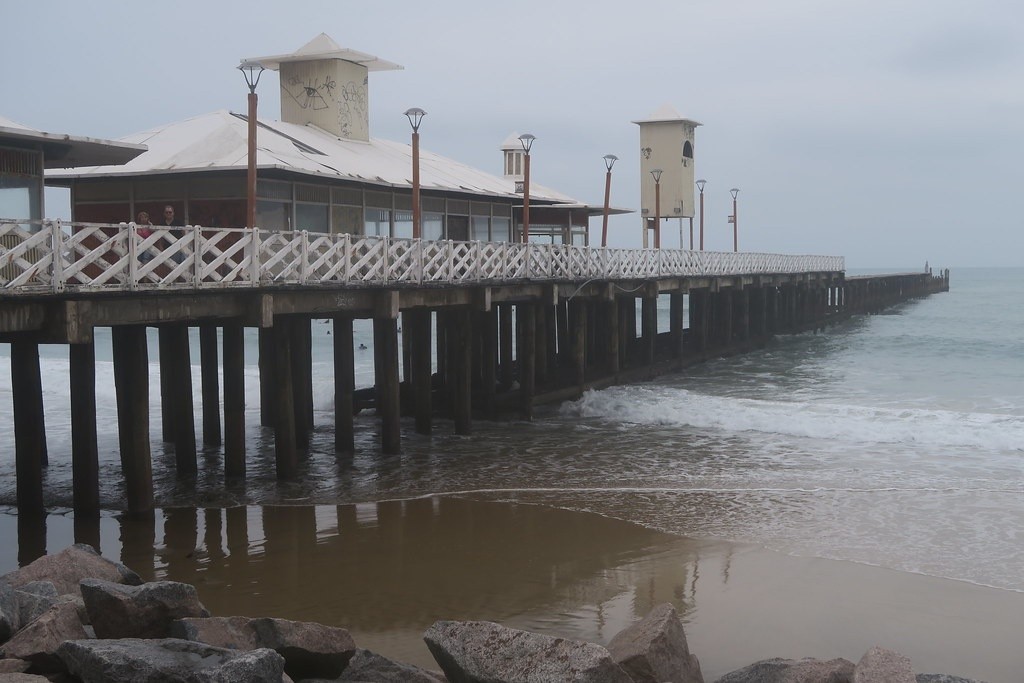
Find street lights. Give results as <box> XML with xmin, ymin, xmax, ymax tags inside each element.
<box><xmin>403</xmin><ymin>107</ymin><xmax>429</xmax><ymax>282</ymax></box>
<box><xmin>695</xmin><ymin>179</ymin><xmax>707</xmax><ymax>273</ymax></box>
<box><xmin>729</xmin><ymin>188</ymin><xmax>741</xmax><ymax>272</ymax></box>
<box><xmin>601</xmin><ymin>154</ymin><xmax>619</xmax><ymax>276</ymax></box>
<box><xmin>650</xmin><ymin>169</ymin><xmax>665</xmax><ymax>274</ymax></box>
<box><xmin>236</xmin><ymin>60</ymin><xmax>266</xmax><ymax>280</ymax></box>
<box><xmin>518</xmin><ymin>133</ymin><xmax>536</xmax><ymax>278</ymax></box>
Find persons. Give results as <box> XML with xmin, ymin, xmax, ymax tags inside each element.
<box><xmin>137</xmin><ymin>212</ymin><xmax>155</xmax><ymax>283</ymax></box>
<box><xmin>159</xmin><ymin>205</ymin><xmax>183</xmax><ymax>282</ymax></box>
